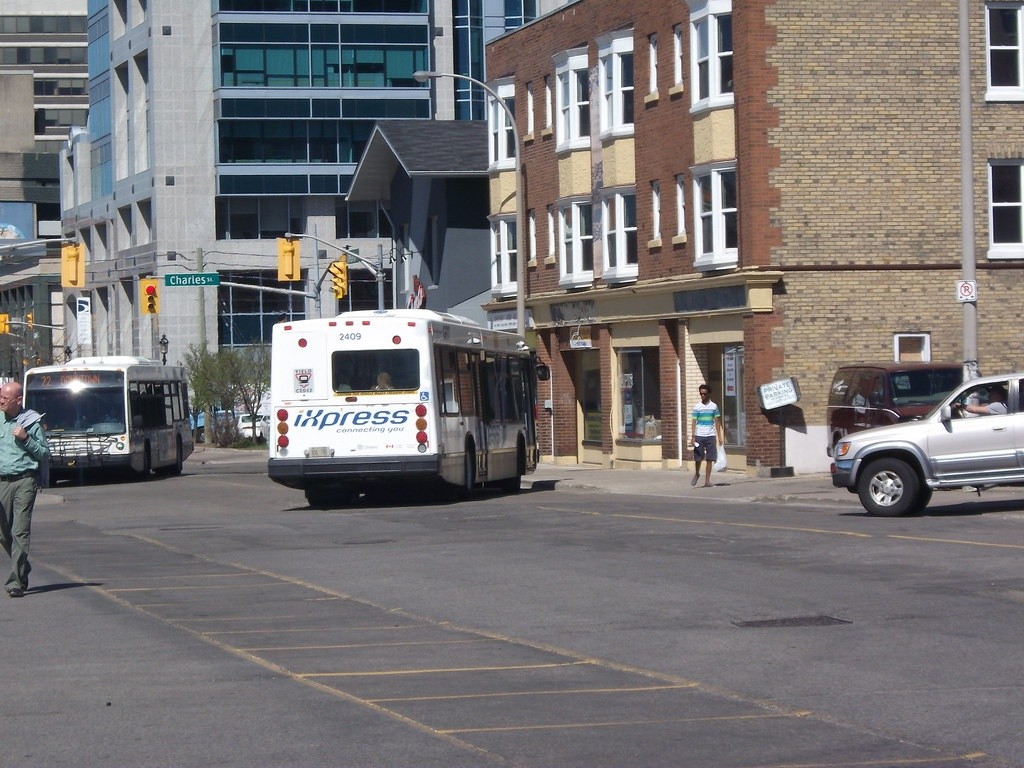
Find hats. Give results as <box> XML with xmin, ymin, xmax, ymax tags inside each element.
<box><xmin>986</xmin><ymin>386</ymin><xmax>1007</xmax><ymax>395</ymax></box>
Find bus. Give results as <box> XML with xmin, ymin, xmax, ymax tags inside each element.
<box><xmin>21</xmin><ymin>353</ymin><xmax>196</xmax><ymax>487</ymax></box>
<box><xmin>267</xmin><ymin>309</ymin><xmax>551</xmax><ymax>509</ymax></box>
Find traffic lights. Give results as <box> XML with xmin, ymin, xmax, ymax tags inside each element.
<box><xmin>25</xmin><ymin>312</ymin><xmax>33</xmax><ymax>329</ymax></box>
<box><xmin>137</xmin><ymin>277</ymin><xmax>159</xmax><ymax>315</ymax></box>
<box><xmin>333</xmin><ymin>261</ymin><xmax>347</xmax><ymax>298</ymax></box>
<box><xmin>327</xmin><ymin>264</ymin><xmax>342</xmax><ymax>298</ymax></box>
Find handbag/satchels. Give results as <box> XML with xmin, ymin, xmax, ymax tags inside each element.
<box><xmin>714</xmin><ymin>444</ymin><xmax>727</xmax><ymax>472</ymax></box>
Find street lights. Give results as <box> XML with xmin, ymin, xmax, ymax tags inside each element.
<box><xmin>282</xmin><ymin>233</ymin><xmax>384</xmax><ymax>311</ymax></box>
<box><xmin>413</xmin><ymin>70</ymin><xmax>525</xmax><ymax>337</ymax></box>
<box><xmin>160</xmin><ymin>332</ymin><xmax>169</xmax><ymax>366</ymax></box>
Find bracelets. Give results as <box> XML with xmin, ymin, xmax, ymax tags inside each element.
<box><xmin>692</xmin><ymin>435</ymin><xmax>696</xmax><ymax>438</ymax></box>
<box><xmin>21</xmin><ymin>434</ymin><xmax>29</xmax><ymax>442</ymax></box>
<box><xmin>960</xmin><ymin>403</ymin><xmax>967</xmax><ymax>409</ymax></box>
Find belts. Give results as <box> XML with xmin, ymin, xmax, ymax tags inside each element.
<box><xmin>0</xmin><ymin>470</ymin><xmax>33</xmax><ymax>482</ymax></box>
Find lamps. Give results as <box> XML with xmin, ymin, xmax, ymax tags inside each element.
<box><xmin>389</xmin><ymin>248</ymin><xmax>402</xmax><ymax>265</ymax></box>
<box><xmin>400</xmin><ymin>246</ymin><xmax>413</xmax><ymax>263</ymax></box>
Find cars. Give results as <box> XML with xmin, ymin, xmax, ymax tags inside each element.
<box><xmin>185</xmin><ymin>409</ymin><xmax>237</xmax><ymax>445</ymax></box>
<box><xmin>231</xmin><ymin>412</ymin><xmax>270</xmax><ymax>441</ymax></box>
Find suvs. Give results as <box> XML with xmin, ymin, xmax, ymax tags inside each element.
<box><xmin>830</xmin><ymin>365</ymin><xmax>1024</xmax><ymax>510</ymax></box>
<box><xmin>826</xmin><ymin>360</ymin><xmax>964</xmax><ymax>461</ymax></box>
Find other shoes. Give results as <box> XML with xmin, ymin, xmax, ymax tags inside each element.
<box><xmin>22</xmin><ymin>564</ymin><xmax>31</xmax><ymax>590</ymax></box>
<box><xmin>691</xmin><ymin>474</ymin><xmax>700</xmax><ymax>486</ymax></box>
<box><xmin>11</xmin><ymin>588</ymin><xmax>23</xmax><ymax>597</ymax></box>
<box><xmin>705</xmin><ymin>483</ymin><xmax>714</xmax><ymax>487</ymax></box>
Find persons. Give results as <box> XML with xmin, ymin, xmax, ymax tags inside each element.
<box><xmin>338</xmin><ymin>371</ymin><xmax>395</xmax><ymax>389</ymax></box>
<box><xmin>953</xmin><ymin>385</ymin><xmax>1008</xmax><ymax>416</ymax></box>
<box><xmin>691</xmin><ymin>385</ymin><xmax>724</xmax><ymax>487</ymax></box>
<box><xmin>0</xmin><ymin>382</ymin><xmax>52</xmax><ymax>597</ymax></box>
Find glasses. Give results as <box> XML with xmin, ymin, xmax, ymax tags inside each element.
<box><xmin>700</xmin><ymin>393</ymin><xmax>706</xmax><ymax>396</ymax></box>
<box><xmin>0</xmin><ymin>396</ymin><xmax>18</xmax><ymax>402</ymax></box>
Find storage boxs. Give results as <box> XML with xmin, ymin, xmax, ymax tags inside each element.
<box><xmin>635</xmin><ymin>417</ymin><xmax>644</xmax><ymax>435</ymax></box>
<box><xmin>625</xmin><ymin>422</ymin><xmax>634</xmax><ymax>433</ymax></box>
<box><xmin>624</xmin><ymin>404</ymin><xmax>633</xmax><ymax>424</ymax></box>
<box><xmin>643</xmin><ymin>421</ymin><xmax>657</xmax><ymax>439</ymax></box>
<box><xmin>655</xmin><ymin>420</ymin><xmax>662</xmax><ymax>436</ymax></box>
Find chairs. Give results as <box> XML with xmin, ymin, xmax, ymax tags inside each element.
<box><xmin>338</xmin><ymin>384</ymin><xmax>352</xmax><ymax>391</ymax></box>
<box><xmin>370</xmin><ymin>371</ymin><xmax>395</xmax><ymax>389</ymax></box>
<box><xmin>908</xmin><ymin>372</ymin><xmax>924</xmax><ymax>393</ymax></box>
